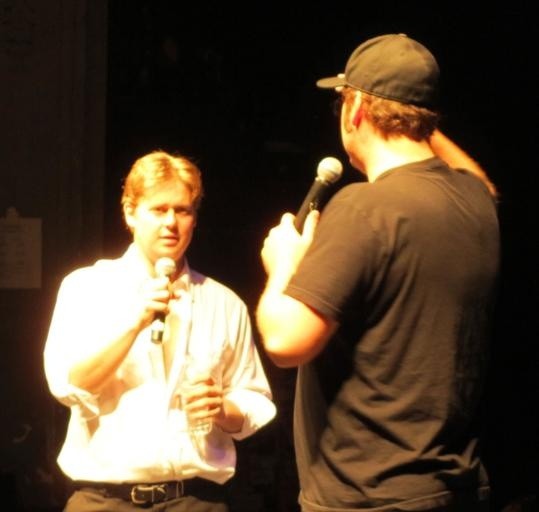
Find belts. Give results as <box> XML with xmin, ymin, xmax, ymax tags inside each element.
<box><xmin>68</xmin><ymin>478</ymin><xmax>196</xmax><ymax>507</ymax></box>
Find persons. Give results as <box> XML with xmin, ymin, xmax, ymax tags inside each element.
<box><xmin>254</xmin><ymin>34</ymin><xmax>511</xmax><ymax>511</ymax></box>
<box><xmin>42</xmin><ymin>152</ymin><xmax>279</xmax><ymax>511</ymax></box>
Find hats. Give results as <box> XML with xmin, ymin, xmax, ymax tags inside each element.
<box><xmin>315</xmin><ymin>33</ymin><xmax>441</xmax><ymax>106</ymax></box>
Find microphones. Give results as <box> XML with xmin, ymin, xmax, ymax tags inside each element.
<box><xmin>150</xmin><ymin>256</ymin><xmax>177</xmax><ymax>344</ymax></box>
<box><xmin>293</xmin><ymin>156</ymin><xmax>344</xmax><ymax>237</ymax></box>
